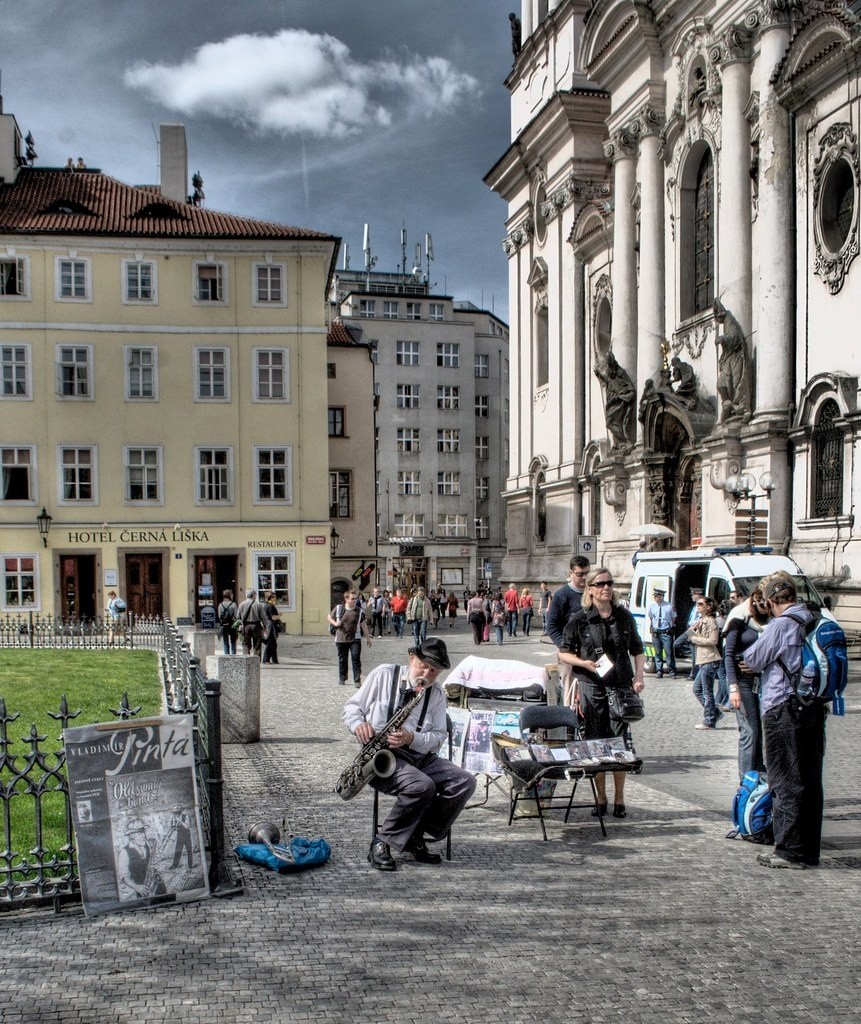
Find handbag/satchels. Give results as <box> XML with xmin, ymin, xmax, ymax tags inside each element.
<box><xmin>483</xmin><ymin>623</ymin><xmax>489</xmax><ymax>642</ymax></box>
<box><xmin>236</xmin><ymin>623</ymin><xmax>245</xmax><ymax>634</ymax></box>
<box><xmin>469</xmin><ymin>612</ymin><xmax>484</xmax><ymax>624</ymax></box>
<box><xmin>606</xmin><ymin>685</ymin><xmax>646</xmax><ymax>723</ymax></box>
<box><xmin>493</xmin><ymin>613</ymin><xmax>505</xmax><ymax>626</ymax></box>
<box><xmin>275</xmin><ymin>623</ymin><xmax>282</xmax><ymax>632</ymax></box>
<box><xmin>115</xmin><ymin>599</ymin><xmax>125</xmax><ymax>613</ymax></box>
<box><xmin>330</xmin><ymin>604</ymin><xmax>345</xmax><ymax>635</ymax></box>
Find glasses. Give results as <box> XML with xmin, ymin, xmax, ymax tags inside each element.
<box><xmin>574</xmin><ymin>571</ymin><xmax>589</xmax><ymax>577</ymax></box>
<box><xmin>589</xmin><ymin>580</ymin><xmax>612</xmax><ymax>587</ymax></box>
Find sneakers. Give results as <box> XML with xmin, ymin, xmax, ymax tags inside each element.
<box><xmin>757</xmin><ymin>852</ymin><xmax>805</xmax><ymax>869</ymax></box>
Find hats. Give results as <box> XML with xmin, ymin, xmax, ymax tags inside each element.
<box><xmin>477</xmin><ymin>721</ymin><xmax>489</xmax><ymax>725</ymax></box>
<box><xmin>246</xmin><ymin>588</ymin><xmax>255</xmax><ymax>598</ymax></box>
<box><xmin>652</xmin><ymin>588</ymin><xmax>666</xmax><ymax>595</ymax></box>
<box><xmin>764</xmin><ymin>579</ymin><xmax>794</xmax><ymax>600</ymax></box>
<box><xmin>408</xmin><ymin>638</ymin><xmax>450</xmax><ymax>669</ymax></box>
<box><xmin>690</xmin><ymin>587</ymin><xmax>703</xmax><ymax>595</ymax></box>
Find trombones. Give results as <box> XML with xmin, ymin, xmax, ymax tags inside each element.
<box><xmin>157</xmin><ymin>817</ymin><xmax>178</xmax><ymax>855</ymax></box>
<box><xmin>247</xmin><ymin>815</ymin><xmax>297</xmax><ymax>866</ymax></box>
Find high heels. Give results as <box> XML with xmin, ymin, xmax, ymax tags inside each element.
<box><xmin>591</xmin><ymin>800</ymin><xmax>627</xmax><ymax>818</ymax></box>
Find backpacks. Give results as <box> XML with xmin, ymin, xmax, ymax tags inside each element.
<box><xmin>219</xmin><ymin>602</ymin><xmax>234</xmax><ymax>627</ymax></box>
<box><xmin>777</xmin><ymin>609</ymin><xmax>848</xmax><ymax>716</ymax></box>
<box><xmin>726</xmin><ymin>769</ymin><xmax>775</xmax><ymax>845</ymax></box>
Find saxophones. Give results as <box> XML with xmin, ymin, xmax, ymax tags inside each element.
<box><xmin>143</xmin><ymin>837</ymin><xmax>165</xmax><ymax>897</ymax></box>
<box><xmin>335</xmin><ymin>683</ymin><xmax>426</xmax><ymax>801</ymax></box>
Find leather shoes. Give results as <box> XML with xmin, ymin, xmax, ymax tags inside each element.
<box><xmin>367</xmin><ymin>841</ymin><xmax>396</xmax><ymax>870</ymax></box>
<box><xmin>405</xmin><ymin>838</ymin><xmax>442</xmax><ymax>864</ymax></box>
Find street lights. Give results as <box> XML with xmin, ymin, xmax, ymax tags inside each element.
<box><xmin>725</xmin><ymin>471</ymin><xmax>781</xmax><ymax>556</ymax></box>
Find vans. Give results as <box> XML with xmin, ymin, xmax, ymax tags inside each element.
<box><xmin>628</xmin><ymin>545</ymin><xmax>840</xmax><ymax>675</ymax></box>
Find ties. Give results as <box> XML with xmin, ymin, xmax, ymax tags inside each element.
<box><xmin>658</xmin><ymin>605</ymin><xmax>661</xmax><ymax>627</ymax></box>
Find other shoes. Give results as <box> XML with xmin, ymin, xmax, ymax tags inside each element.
<box><xmin>261</xmin><ymin>658</ymin><xmax>279</xmax><ymax>665</ymax></box>
<box><xmin>657</xmin><ymin>671</ymin><xmax>678</xmax><ymax>679</ymax></box>
<box><xmin>697</xmin><ymin>706</ymin><xmax>729</xmax><ymax>729</ymax></box>
<box><xmin>340</xmin><ymin>680</ymin><xmax>361</xmax><ymax>688</ymax></box>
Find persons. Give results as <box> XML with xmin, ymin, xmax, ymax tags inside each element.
<box><xmin>638</xmin><ymin>379</ymin><xmax>655</xmax><ymax>419</ymax></box>
<box><xmin>342</xmin><ymin>636</ymin><xmax>476</xmax><ymax>870</ymax></box>
<box><xmin>557</xmin><ymin>568</ymin><xmax>645</xmax><ymax>818</ymax></box>
<box><xmin>104</xmin><ymin>590</ymin><xmax>129</xmax><ymax>644</ymax></box>
<box><xmin>648</xmin><ymin>589</ymin><xmax>677</xmax><ymax>677</ymax></box>
<box><xmin>65</xmin><ymin>157</ymin><xmax>86</xmax><ymax>168</ymax></box>
<box><xmin>463</xmin><ymin>582</ymin><xmax>534</xmax><ymax>646</ymax></box>
<box><xmin>358</xmin><ymin>584</ymin><xmax>458</xmax><ymax>645</ymax></box>
<box><xmin>660</xmin><ymin>357</ymin><xmax>697</xmax><ymax>410</ymax></box>
<box><xmin>594</xmin><ymin>353</ymin><xmax>636</xmax><ymax>450</ymax></box>
<box><xmin>742</xmin><ymin>579</ymin><xmax>826</xmax><ymax>868</ymax></box>
<box><xmin>686</xmin><ymin>587</ymin><xmax>746</xmax><ymax>730</ymax></box>
<box><xmin>449</xmin><ymin>715</ymin><xmax>619</xmax><ymax>769</ymax></box>
<box><xmin>723</xmin><ymin>571</ymin><xmax>793</xmax><ymax>781</ymax></box>
<box><xmin>546</xmin><ymin>556</ymin><xmax>590</xmax><ymax>707</ymax></box>
<box><xmin>509</xmin><ymin>13</ymin><xmax>521</xmax><ymax>59</ymax></box>
<box><xmin>327</xmin><ymin>590</ymin><xmax>372</xmax><ymax>689</ymax></box>
<box><xmin>218</xmin><ymin>590</ymin><xmax>282</xmax><ymax>665</ymax></box>
<box><xmin>710</xmin><ymin>297</ymin><xmax>750</xmax><ymax>415</ymax></box>
<box><xmin>538</xmin><ymin>582</ymin><xmax>552</xmax><ymax>636</ymax></box>
